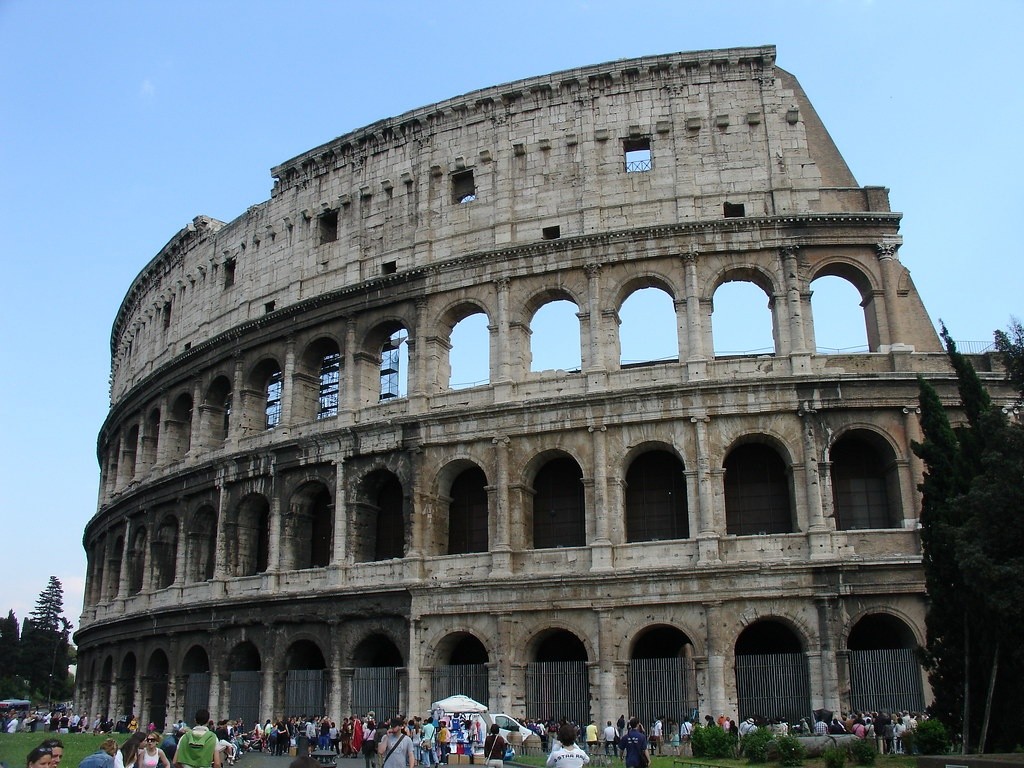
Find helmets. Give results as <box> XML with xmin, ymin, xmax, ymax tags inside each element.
<box><xmin>368</xmin><ymin>711</ymin><xmax>375</xmax><ymax>718</ymax></box>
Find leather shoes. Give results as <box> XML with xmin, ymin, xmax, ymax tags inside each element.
<box><xmin>274</xmin><ymin>753</ymin><xmax>279</xmax><ymax>756</ymax></box>
<box><xmin>279</xmin><ymin>753</ymin><xmax>282</xmax><ymax>756</ymax></box>
<box><xmin>435</xmin><ymin>763</ymin><xmax>439</xmax><ymax>768</ymax></box>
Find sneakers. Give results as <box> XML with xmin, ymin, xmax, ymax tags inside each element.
<box><xmin>229</xmin><ymin>762</ymin><xmax>234</xmax><ymax>766</ymax></box>
<box><xmin>444</xmin><ymin>762</ymin><xmax>448</xmax><ymax>765</ymax></box>
<box><xmin>228</xmin><ymin>755</ymin><xmax>234</xmax><ymax>758</ymax></box>
<box><xmin>439</xmin><ymin>763</ymin><xmax>444</xmax><ymax>765</ymax></box>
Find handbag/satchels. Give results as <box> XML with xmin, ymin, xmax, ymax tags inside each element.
<box><xmin>659</xmin><ymin>740</ymin><xmax>664</xmax><ymax>745</ymax></box>
<box><xmin>361</xmin><ymin>740</ymin><xmax>369</xmax><ymax>753</ymax></box>
<box><xmin>613</xmin><ymin>735</ymin><xmax>620</xmax><ymax>746</ymax></box>
<box><xmin>852</xmin><ymin>731</ymin><xmax>856</xmax><ymax>735</ymax></box>
<box><xmin>638</xmin><ymin>749</ymin><xmax>649</xmax><ymax>768</ymax></box>
<box><xmin>864</xmin><ymin>730</ymin><xmax>869</xmax><ymax>737</ymax></box>
<box><xmin>484</xmin><ymin>757</ymin><xmax>490</xmax><ymax>766</ymax></box>
<box><xmin>686</xmin><ymin>734</ymin><xmax>693</xmax><ymax>743</ymax></box>
<box><xmin>420</xmin><ymin>739</ymin><xmax>432</xmax><ymax>750</ymax></box>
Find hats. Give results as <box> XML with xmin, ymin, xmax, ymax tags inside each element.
<box><xmin>800</xmin><ymin>718</ymin><xmax>805</xmax><ymax>722</ymax></box>
<box><xmin>746</xmin><ymin>718</ymin><xmax>755</xmax><ymax>724</ymax></box>
<box><xmin>669</xmin><ymin>735</ymin><xmax>673</xmax><ymax>742</ymax></box>
<box><xmin>682</xmin><ymin>734</ymin><xmax>687</xmax><ymax>742</ymax></box>
<box><xmin>390</xmin><ymin>718</ymin><xmax>404</xmax><ymax>726</ymax></box>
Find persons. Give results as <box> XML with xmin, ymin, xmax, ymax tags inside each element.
<box><xmin>852</xmin><ymin>709</ymin><xmax>929</xmax><ymax>756</ymax></box>
<box><xmin>739</xmin><ymin>709</ymin><xmax>852</xmax><ymax>755</ymax></box>
<box><xmin>92</xmin><ymin>709</ymin><xmax>157</xmax><ymax>732</ymax></box>
<box><xmin>361</xmin><ymin>709</ymin><xmax>450</xmax><ymax>768</ymax></box>
<box><xmin>484</xmin><ymin>724</ymin><xmax>506</xmax><ymax>768</ymax></box>
<box><xmin>546</xmin><ymin>724</ymin><xmax>590</xmax><ymax>768</ymax></box>
<box><xmin>264</xmin><ymin>712</ymin><xmax>361</xmax><ymax>759</ymax></box>
<box><xmin>670</xmin><ymin>712</ymin><xmax>739</xmax><ymax>760</ymax></box>
<box><xmin>77</xmin><ymin>732</ymin><xmax>171</xmax><ymax>768</ymax></box>
<box><xmin>25</xmin><ymin>745</ymin><xmax>52</xmax><ymax>768</ymax></box>
<box><xmin>172</xmin><ymin>708</ymin><xmax>264</xmax><ymax>768</ymax></box>
<box><xmin>2</xmin><ymin>705</ymin><xmax>89</xmax><ymax>738</ymax></box>
<box><xmin>586</xmin><ymin>713</ymin><xmax>666</xmax><ymax>768</ymax></box>
<box><xmin>512</xmin><ymin>715</ymin><xmax>585</xmax><ymax>757</ymax></box>
<box><xmin>41</xmin><ymin>739</ymin><xmax>67</xmax><ymax>768</ymax></box>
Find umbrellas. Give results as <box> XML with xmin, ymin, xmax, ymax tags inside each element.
<box><xmin>428</xmin><ymin>694</ymin><xmax>488</xmax><ymax>713</ymax></box>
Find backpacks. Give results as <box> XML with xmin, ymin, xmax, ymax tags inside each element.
<box><xmin>550</xmin><ymin>725</ymin><xmax>557</xmax><ymax>732</ymax></box>
<box><xmin>452</xmin><ymin>718</ymin><xmax>460</xmax><ymax>730</ymax></box>
<box><xmin>527</xmin><ymin>724</ymin><xmax>534</xmax><ymax>731</ymax></box>
<box><xmin>464</xmin><ymin>720</ymin><xmax>472</xmax><ymax>730</ymax></box>
<box><xmin>535</xmin><ymin>726</ymin><xmax>542</xmax><ymax>734</ymax></box>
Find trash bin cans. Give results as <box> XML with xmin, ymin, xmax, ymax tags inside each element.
<box><xmin>298</xmin><ymin>731</ymin><xmax>309</xmax><ymax>754</ymax></box>
<box><xmin>307</xmin><ymin>750</ymin><xmax>338</xmax><ymax>768</ymax></box>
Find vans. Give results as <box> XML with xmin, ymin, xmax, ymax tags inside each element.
<box><xmin>445</xmin><ymin>712</ymin><xmax>539</xmax><ymax>743</ymax></box>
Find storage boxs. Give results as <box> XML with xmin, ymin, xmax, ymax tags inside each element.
<box><xmin>473</xmin><ymin>753</ymin><xmax>484</xmax><ymax>765</ymax></box>
<box><xmin>448</xmin><ymin>754</ymin><xmax>458</xmax><ymax>765</ymax></box>
<box><xmin>459</xmin><ymin>755</ymin><xmax>470</xmax><ymax>765</ymax></box>
<box><xmin>289</xmin><ymin>747</ymin><xmax>298</xmax><ymax>757</ymax></box>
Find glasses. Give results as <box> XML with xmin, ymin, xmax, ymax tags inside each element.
<box><xmin>50</xmin><ymin>741</ymin><xmax>62</xmax><ymax>746</ymax></box>
<box><xmin>145</xmin><ymin>738</ymin><xmax>159</xmax><ymax>743</ymax></box>
<box><xmin>37</xmin><ymin>747</ymin><xmax>52</xmax><ymax>752</ymax></box>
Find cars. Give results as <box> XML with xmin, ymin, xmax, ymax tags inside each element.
<box><xmin>51</xmin><ymin>703</ymin><xmax>67</xmax><ymax>715</ymax></box>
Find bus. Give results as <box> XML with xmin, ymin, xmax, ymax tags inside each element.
<box><xmin>0</xmin><ymin>699</ymin><xmax>32</xmax><ymax>717</ymax></box>
